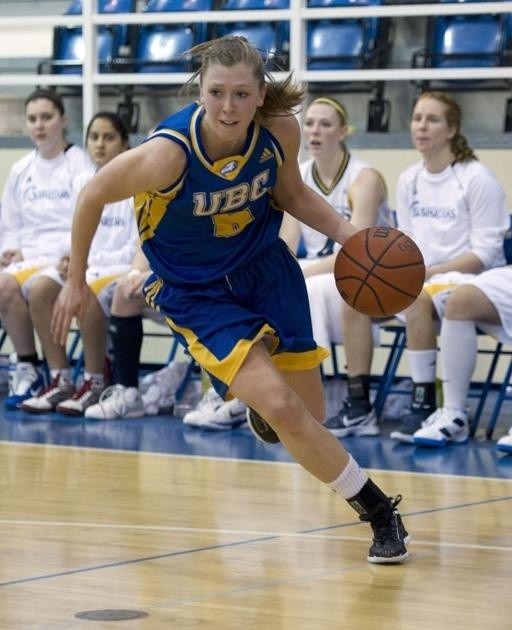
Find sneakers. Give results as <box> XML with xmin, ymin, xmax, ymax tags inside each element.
<box><xmin>324</xmin><ymin>402</ymin><xmax>381</xmax><ymax>438</ymax></box>
<box><xmin>84</xmin><ymin>384</ymin><xmax>144</xmax><ymax>419</ymax></box>
<box><xmin>246</xmin><ymin>405</ymin><xmax>281</xmax><ymax>444</ymax></box>
<box><xmin>413</xmin><ymin>408</ymin><xmax>470</xmax><ymax>447</ymax></box>
<box><xmin>359</xmin><ymin>495</ymin><xmax>411</xmax><ymax>564</ymax></box>
<box><xmin>0</xmin><ymin>354</ymin><xmax>10</xmax><ymax>395</ymax></box>
<box><xmin>4</xmin><ymin>362</ymin><xmax>49</xmax><ymax>407</ymax></box>
<box><xmin>496</xmin><ymin>427</ymin><xmax>512</xmax><ymax>452</ymax></box>
<box><xmin>183</xmin><ymin>387</ymin><xmax>225</xmax><ymax>426</ymax></box>
<box><xmin>22</xmin><ymin>373</ymin><xmax>76</xmax><ymax>413</ymax></box>
<box><xmin>56</xmin><ymin>376</ymin><xmax>106</xmax><ymax>417</ymax></box>
<box><xmin>199</xmin><ymin>397</ymin><xmax>247</xmax><ymax>430</ymax></box>
<box><xmin>174</xmin><ymin>380</ymin><xmax>203</xmax><ymax>418</ymax></box>
<box><xmin>390</xmin><ymin>405</ymin><xmax>442</xmax><ymax>444</ymax></box>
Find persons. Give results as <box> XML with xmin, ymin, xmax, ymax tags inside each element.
<box><xmin>0</xmin><ymin>90</ymin><xmax>96</xmax><ymax>408</ymax></box>
<box><xmin>205</xmin><ymin>97</ymin><xmax>395</xmax><ymax>429</ymax></box>
<box><xmin>22</xmin><ymin>111</ymin><xmax>139</xmax><ymax>414</ymax></box>
<box><xmin>50</xmin><ymin>38</ymin><xmax>410</xmax><ymax>563</ymax></box>
<box><xmin>321</xmin><ymin>92</ymin><xmax>510</xmax><ymax>443</ymax></box>
<box><xmin>85</xmin><ymin>267</ymin><xmax>223</xmax><ymax>426</ymax></box>
<box><xmin>414</xmin><ymin>263</ymin><xmax>512</xmax><ymax>450</ymax></box>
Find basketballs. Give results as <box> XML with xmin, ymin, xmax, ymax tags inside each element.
<box><xmin>334</xmin><ymin>226</ymin><xmax>426</xmax><ymax>319</ymax></box>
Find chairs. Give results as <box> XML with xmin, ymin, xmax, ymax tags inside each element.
<box><xmin>306</xmin><ymin>0</ymin><xmax>395</xmax><ymax>133</ymax></box>
<box><xmin>410</xmin><ymin>0</ymin><xmax>512</xmax><ymax>134</ymax></box>
<box><xmin>36</xmin><ymin>0</ymin><xmax>126</xmax><ymax>132</ymax></box>
<box><xmin>376</xmin><ymin>214</ymin><xmax>512</xmax><ymax>436</ymax></box>
<box><xmin>217</xmin><ymin>0</ymin><xmax>290</xmax><ymax>72</ymax></box>
<box><xmin>127</xmin><ymin>0</ymin><xmax>214</xmax><ymax>133</ymax></box>
